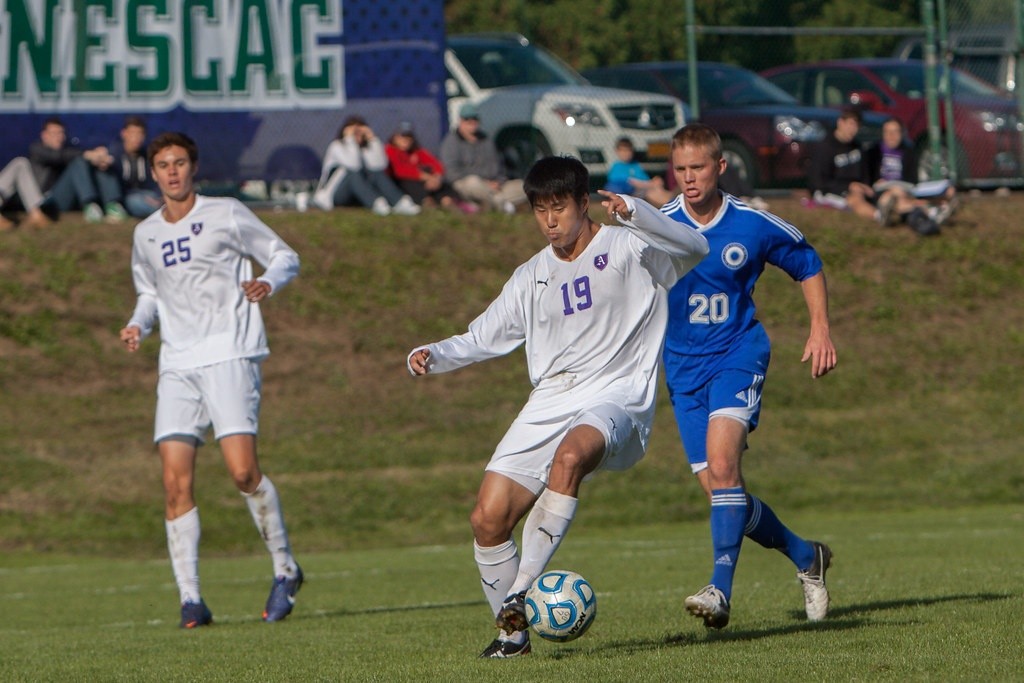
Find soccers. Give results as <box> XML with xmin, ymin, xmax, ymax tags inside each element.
<box><xmin>524</xmin><ymin>570</ymin><xmax>599</xmax><ymax>643</ymax></box>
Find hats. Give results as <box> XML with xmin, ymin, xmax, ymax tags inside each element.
<box><xmin>399</xmin><ymin>121</ymin><xmax>414</xmax><ymax>135</ymax></box>
<box><xmin>460</xmin><ymin>104</ymin><xmax>480</xmax><ymax>119</ymax></box>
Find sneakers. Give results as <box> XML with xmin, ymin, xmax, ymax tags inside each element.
<box><xmin>495</xmin><ymin>590</ymin><xmax>530</xmax><ymax>635</ymax></box>
<box><xmin>263</xmin><ymin>560</ymin><xmax>304</xmax><ymax>623</ymax></box>
<box><xmin>478</xmin><ymin>631</ymin><xmax>531</xmax><ymax>658</ymax></box>
<box><xmin>686</xmin><ymin>584</ymin><xmax>731</xmax><ymax>629</ymax></box>
<box><xmin>180</xmin><ymin>598</ymin><xmax>210</xmax><ymax>628</ymax></box>
<box><xmin>796</xmin><ymin>540</ymin><xmax>833</xmax><ymax>622</ymax></box>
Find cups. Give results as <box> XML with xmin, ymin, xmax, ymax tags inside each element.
<box><xmin>297</xmin><ymin>192</ymin><xmax>309</xmax><ymax>211</ymax></box>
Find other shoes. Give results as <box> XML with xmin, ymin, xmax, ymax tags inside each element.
<box><xmin>460</xmin><ymin>199</ymin><xmax>480</xmax><ymax>213</ymax></box>
<box><xmin>373</xmin><ymin>195</ymin><xmax>392</xmax><ymax>216</ymax></box>
<box><xmin>502</xmin><ymin>202</ymin><xmax>517</xmax><ymax>215</ymax></box>
<box><xmin>84</xmin><ymin>202</ymin><xmax>103</xmax><ymax>224</ymax></box>
<box><xmin>753</xmin><ymin>196</ymin><xmax>768</xmax><ymax>210</ymax></box>
<box><xmin>426</xmin><ymin>204</ymin><xmax>445</xmax><ymax>216</ymax></box>
<box><xmin>104</xmin><ymin>201</ymin><xmax>126</xmax><ymax>222</ymax></box>
<box><xmin>394</xmin><ymin>194</ymin><xmax>421</xmax><ymax>215</ymax></box>
<box><xmin>877</xmin><ymin>195</ymin><xmax>896</xmax><ymax>227</ymax></box>
<box><xmin>930</xmin><ymin>196</ymin><xmax>958</xmax><ymax>223</ymax></box>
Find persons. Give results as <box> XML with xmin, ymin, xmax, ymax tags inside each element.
<box><xmin>119</xmin><ymin>131</ymin><xmax>304</xmax><ymax>627</ymax></box>
<box><xmin>314</xmin><ymin>115</ymin><xmax>420</xmax><ymax>216</ymax></box>
<box><xmin>110</xmin><ymin>115</ymin><xmax>163</xmax><ymax>217</ymax></box>
<box><xmin>0</xmin><ymin>156</ymin><xmax>51</xmax><ymax>232</ymax></box>
<box><xmin>815</xmin><ymin>108</ymin><xmax>956</xmax><ymax>227</ymax></box>
<box><xmin>657</xmin><ymin>123</ymin><xmax>838</xmax><ymax>631</ymax></box>
<box><xmin>28</xmin><ymin>117</ymin><xmax>127</xmax><ymax>225</ymax></box>
<box><xmin>440</xmin><ymin>104</ymin><xmax>528</xmax><ymax>213</ymax></box>
<box><xmin>603</xmin><ymin>138</ymin><xmax>673</xmax><ymax>209</ymax></box>
<box><xmin>407</xmin><ymin>156</ymin><xmax>710</xmax><ymax>658</ymax></box>
<box><xmin>384</xmin><ymin>121</ymin><xmax>454</xmax><ymax>207</ymax></box>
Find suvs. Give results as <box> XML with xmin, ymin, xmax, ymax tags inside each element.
<box><xmin>268</xmin><ymin>35</ymin><xmax>687</xmax><ymax>186</ymax></box>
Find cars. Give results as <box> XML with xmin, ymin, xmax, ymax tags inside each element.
<box><xmin>581</xmin><ymin>58</ymin><xmax>918</xmax><ymax>201</ymax></box>
<box><xmin>757</xmin><ymin>58</ymin><xmax>1023</xmax><ymax>193</ymax></box>
